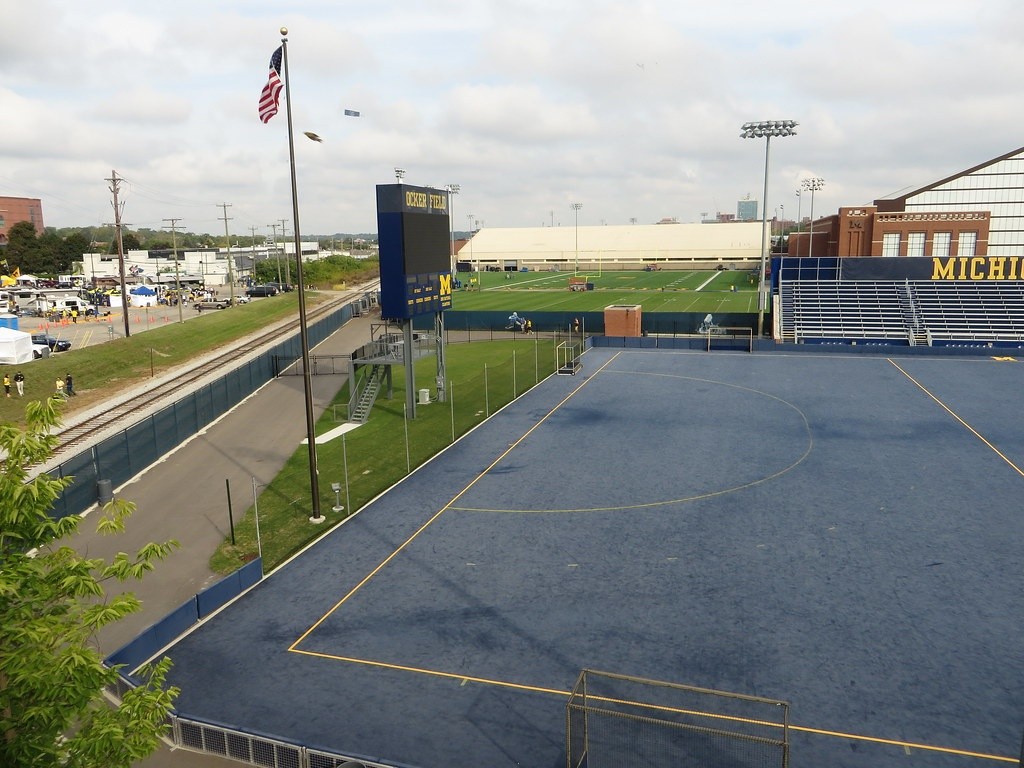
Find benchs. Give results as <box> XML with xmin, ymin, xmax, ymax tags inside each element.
<box><xmin>794</xmin><ymin>279</ymin><xmax>1024</xmax><ymax>340</ymax></box>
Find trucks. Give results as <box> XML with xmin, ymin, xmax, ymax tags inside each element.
<box><xmin>645</xmin><ymin>264</ymin><xmax>663</xmax><ymax>272</ymax></box>
<box><xmin>8</xmin><ymin>290</ymin><xmax>48</xmax><ymax>313</ymax></box>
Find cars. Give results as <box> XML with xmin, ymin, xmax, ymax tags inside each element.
<box><xmin>32</xmin><ymin>334</ymin><xmax>71</xmax><ymax>352</ymax></box>
<box><xmin>224</xmin><ymin>295</ymin><xmax>249</xmax><ymax>303</ymax></box>
<box><xmin>186</xmin><ymin>286</ymin><xmax>214</xmax><ymax>295</ymax></box>
<box><xmin>238</xmin><ymin>275</ymin><xmax>247</xmax><ymax>282</ymax></box>
<box><xmin>29</xmin><ymin>341</ymin><xmax>52</xmax><ymax>359</ymax></box>
<box><xmin>40</xmin><ymin>279</ymin><xmax>54</xmax><ymax>288</ymax></box>
<box><xmin>54</xmin><ymin>282</ymin><xmax>71</xmax><ymax>289</ymax></box>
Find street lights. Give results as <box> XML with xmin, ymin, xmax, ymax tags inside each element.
<box><xmin>739</xmin><ymin>117</ymin><xmax>798</xmax><ymax>338</ymax></box>
<box><xmin>779</xmin><ymin>203</ymin><xmax>786</xmax><ymax>256</ymax></box>
<box><xmin>443</xmin><ymin>182</ymin><xmax>461</xmax><ymax>285</ymax></box>
<box><xmin>796</xmin><ymin>188</ymin><xmax>801</xmax><ymax>256</ymax></box>
<box><xmin>567</xmin><ymin>199</ymin><xmax>582</xmax><ymax>270</ymax></box>
<box><xmin>802</xmin><ymin>175</ymin><xmax>819</xmax><ymax>257</ymax></box>
<box><xmin>394</xmin><ymin>166</ymin><xmax>406</xmax><ymax>183</ymax></box>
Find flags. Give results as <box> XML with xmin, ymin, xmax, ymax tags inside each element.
<box><xmin>0</xmin><ymin>259</ymin><xmax>6</xmax><ymax>265</ymax></box>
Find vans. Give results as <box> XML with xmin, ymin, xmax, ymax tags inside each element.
<box><xmin>271</xmin><ymin>283</ymin><xmax>292</xmax><ymax>292</ymax></box>
<box><xmin>53</xmin><ymin>296</ymin><xmax>94</xmax><ymax>314</ymax></box>
<box><xmin>245</xmin><ymin>286</ymin><xmax>278</xmax><ymax>298</ymax></box>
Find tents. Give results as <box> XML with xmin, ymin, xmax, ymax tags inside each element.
<box><xmin>132</xmin><ymin>286</ymin><xmax>155</xmax><ymax>296</ymax></box>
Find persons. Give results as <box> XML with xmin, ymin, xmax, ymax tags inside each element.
<box><xmin>526</xmin><ymin>320</ymin><xmax>534</xmax><ymax>335</ymax></box>
<box><xmin>160</xmin><ymin>285</ymin><xmax>251</xmax><ymax>315</ymax></box>
<box><xmin>3</xmin><ymin>374</ymin><xmax>12</xmax><ymax>397</ymax></box>
<box><xmin>9</xmin><ymin>279</ymin><xmax>131</xmax><ymax>323</ymax></box>
<box><xmin>574</xmin><ymin>318</ymin><xmax>579</xmax><ymax>334</ymax></box>
<box><xmin>465</xmin><ymin>281</ymin><xmax>475</xmax><ymax>291</ymax></box>
<box><xmin>56</xmin><ymin>377</ymin><xmax>64</xmax><ymax>393</ymax></box>
<box><xmin>65</xmin><ymin>374</ymin><xmax>75</xmax><ymax>397</ymax></box>
<box><xmin>14</xmin><ymin>371</ymin><xmax>24</xmax><ymax>397</ymax></box>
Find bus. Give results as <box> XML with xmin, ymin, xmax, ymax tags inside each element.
<box><xmin>165</xmin><ymin>289</ymin><xmax>195</xmax><ymax>303</ymax></box>
<box><xmin>59</xmin><ymin>275</ymin><xmax>88</xmax><ymax>286</ymax></box>
<box><xmin>114</xmin><ymin>285</ymin><xmax>169</xmax><ymax>299</ymax></box>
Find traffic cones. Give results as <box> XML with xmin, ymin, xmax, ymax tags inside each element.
<box><xmin>61</xmin><ymin>319</ymin><xmax>64</xmax><ymax>325</ymax></box>
<box><xmin>53</xmin><ymin>319</ymin><xmax>58</xmax><ymax>327</ymax></box>
<box><xmin>39</xmin><ymin>322</ymin><xmax>44</xmax><ymax>331</ymax></box>
<box><xmin>45</xmin><ymin>321</ymin><xmax>50</xmax><ymax>329</ymax></box>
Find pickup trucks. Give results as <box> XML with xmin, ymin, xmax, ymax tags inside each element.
<box><xmin>194</xmin><ymin>297</ymin><xmax>227</xmax><ymax>310</ymax></box>
<box><xmin>716</xmin><ymin>265</ymin><xmax>729</xmax><ymax>271</ymax></box>
